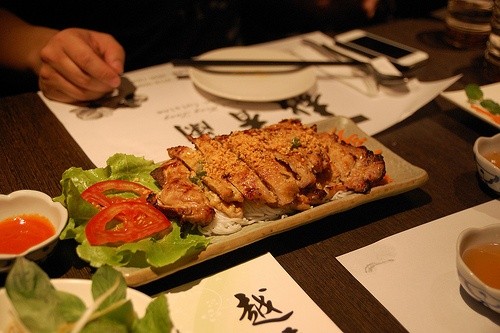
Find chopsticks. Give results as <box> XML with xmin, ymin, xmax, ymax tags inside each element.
<box><xmin>170</xmin><ymin>56</ymin><xmax>365</xmax><ymax>70</ymax></box>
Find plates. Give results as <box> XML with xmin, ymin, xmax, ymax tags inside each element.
<box><xmin>79</xmin><ymin>118</ymin><xmax>428</xmax><ymax>286</ymax></box>
<box><xmin>190</xmin><ymin>43</ymin><xmax>319</xmax><ymax>102</ymax></box>
<box><xmin>441</xmin><ymin>83</ymin><xmax>500</xmax><ymax>129</ymax></box>
<box><xmin>0</xmin><ymin>278</ymin><xmax>175</xmax><ymax>333</ymax></box>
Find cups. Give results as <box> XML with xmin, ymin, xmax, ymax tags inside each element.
<box><xmin>486</xmin><ymin>0</ymin><xmax>500</xmax><ymax>65</ymax></box>
<box><xmin>446</xmin><ymin>0</ymin><xmax>493</xmax><ymax>47</ymax></box>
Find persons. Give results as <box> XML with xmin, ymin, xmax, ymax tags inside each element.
<box><xmin>0</xmin><ymin>0</ymin><xmax>370</xmax><ymax>104</ymax></box>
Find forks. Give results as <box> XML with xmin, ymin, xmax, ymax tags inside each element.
<box><xmin>322</xmin><ymin>44</ymin><xmax>414</xmax><ymax>86</ymax></box>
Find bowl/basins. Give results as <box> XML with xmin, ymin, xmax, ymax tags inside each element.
<box><xmin>472</xmin><ymin>132</ymin><xmax>500</xmax><ymax>192</ymax></box>
<box><xmin>454</xmin><ymin>223</ymin><xmax>500</xmax><ymax>316</ymax></box>
<box><xmin>0</xmin><ymin>189</ymin><xmax>68</xmax><ymax>272</ymax></box>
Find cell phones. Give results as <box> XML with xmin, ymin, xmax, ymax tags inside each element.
<box><xmin>334</xmin><ymin>29</ymin><xmax>430</xmax><ymax>69</ymax></box>
